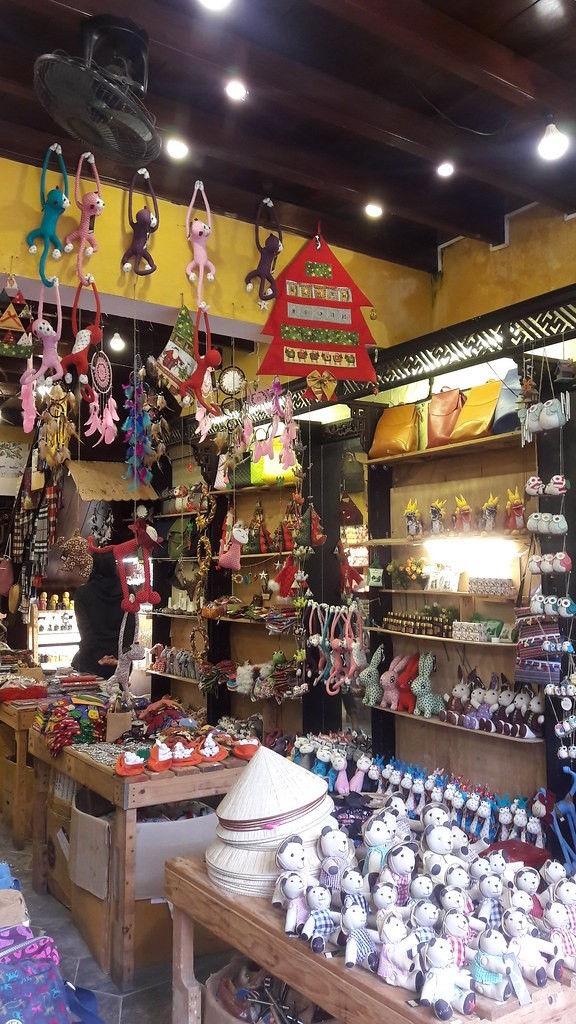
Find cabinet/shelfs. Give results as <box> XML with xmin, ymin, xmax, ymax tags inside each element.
<box><xmin>0</xmin><ymin>295</ymin><xmax>576</xmax><ymax>1024</ymax></box>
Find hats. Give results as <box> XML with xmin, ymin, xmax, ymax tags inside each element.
<box><xmin>204</xmin><ymin>746</ymin><xmax>341</xmax><ymax>901</ymax></box>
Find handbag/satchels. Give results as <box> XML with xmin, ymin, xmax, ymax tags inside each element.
<box><xmin>339</xmin><ymin>493</ymin><xmax>365</xmax><ymax>525</ymax></box>
<box><xmin>0</xmin><ymin>533</ymin><xmax>14</xmax><ymax>597</ymax></box>
<box><xmin>339</xmin><ymin>451</ymin><xmax>365</xmax><ymax>491</ymax></box>
<box><xmin>450</xmin><ymin>379</ymin><xmax>503</xmax><ymax>440</ymax></box>
<box><xmin>214</xmin><ymin>423</ymin><xmax>303</xmax><ymax>490</ymax></box>
<box><xmin>369</xmin><ymin>402</ymin><xmax>423</xmax><ymax>458</ymax></box>
<box><xmin>492</xmin><ymin>369</ymin><xmax>522</xmax><ymax>434</ymax></box>
<box><xmin>427</xmin><ymin>385</ymin><xmax>467</xmax><ymax>448</ymax></box>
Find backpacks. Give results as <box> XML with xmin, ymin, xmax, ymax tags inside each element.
<box><xmin>554</xmin><ymin>358</ymin><xmax>575</xmax><ymax>384</ymax></box>
<box><xmin>151</xmin><ymin>516</ymin><xmax>209</xmax><ymax>558</ymax></box>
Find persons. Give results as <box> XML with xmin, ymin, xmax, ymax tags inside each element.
<box><xmin>71</xmin><ymin>553</ymin><xmax>137</xmax><ymax>687</ymax></box>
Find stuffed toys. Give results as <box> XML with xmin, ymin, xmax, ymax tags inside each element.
<box><xmin>518</xmin><ymin>392</ymin><xmax>576</xmax><ymax>787</ymax></box>
<box><xmin>309</xmin><ymin>602</ymin><xmax>544</xmax><ymax>739</ymax></box>
<box><xmin>20</xmin><ymin>144</ymin><xmax>284</xmax><ymax>415</ymax></box>
<box><xmin>271</xmin><ymin>787</ymin><xmax>576</xmax><ymax>1024</ymax></box>
<box><xmin>149</xmin><ymin>642</ymin><xmax>207</xmax><ymax>679</ymax></box>
<box><xmin>291</xmin><ymin>727</ymin><xmax>547</xmax><ymax>849</ymax></box>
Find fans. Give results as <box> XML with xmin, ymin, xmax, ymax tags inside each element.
<box><xmin>32</xmin><ymin>13</ymin><xmax>163</xmax><ymax>169</ymax></box>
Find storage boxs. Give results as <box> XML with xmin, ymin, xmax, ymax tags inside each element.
<box><xmin>45</xmin><ymin>764</ymin><xmax>80</xmax><ymax>911</ymax></box>
<box><xmin>71</xmin><ymin>785</ymin><xmax>234</xmax><ymax>973</ymax></box>
<box><xmin>0</xmin><ymin>723</ymin><xmax>37</xmax><ymax>835</ymax></box>
<box><xmin>203</xmin><ymin>957</ymin><xmax>319</xmax><ymax>1024</ymax></box>
